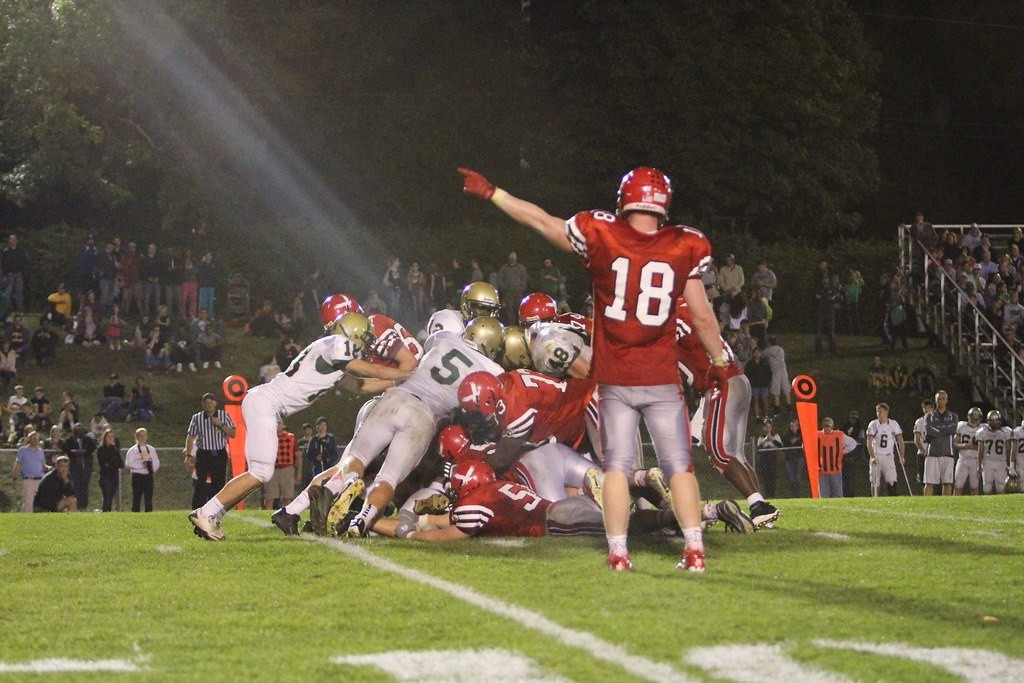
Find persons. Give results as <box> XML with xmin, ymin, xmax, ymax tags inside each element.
<box><xmin>817</xmin><ymin>389</ymin><xmax>1022</xmax><ymax>499</ymax></box>
<box><xmin>910</xmin><ymin>212</ymin><xmax>1024</xmax><ymax>420</ymax></box>
<box><xmin>0</xmin><ymin>234</ymin><xmax>322</xmax><ymax>388</ymax></box>
<box><xmin>271</xmin><ymin>281</ymin><xmax>781</xmax><ymax>535</ymax></box>
<box><xmin>700</xmin><ymin>253</ymin><xmax>777</xmax><ymax>307</ymax></box>
<box><xmin>365</xmin><ymin>250</ymin><xmax>571</xmax><ymax>315</ymax></box>
<box><xmin>762</xmin><ymin>335</ymin><xmax>792</xmax><ymax>406</ymax></box>
<box><xmin>182</xmin><ymin>393</ymin><xmax>237</xmax><ymax>510</ymax></box>
<box><xmin>457</xmin><ymin>167</ymin><xmax>734</xmax><ymax>573</ymax></box>
<box><xmin>264</xmin><ymin>415</ymin><xmax>339</xmax><ymax>510</ymax></box>
<box><xmin>0</xmin><ymin>373</ymin><xmax>161</xmax><ymax>513</ymax></box>
<box><xmin>756</xmin><ymin>419</ymin><xmax>805</xmax><ymax>498</ymax></box>
<box><xmin>188</xmin><ymin>312</ymin><xmax>415</xmax><ymax>541</ymax></box>
<box><xmin>811</xmin><ymin>259</ymin><xmax>936</xmax><ymax>396</ymax></box>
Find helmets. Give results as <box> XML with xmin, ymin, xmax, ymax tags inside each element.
<box><xmin>435</xmin><ymin>422</ymin><xmax>471</xmax><ymax>465</ymax></box>
<box><xmin>986</xmin><ymin>410</ymin><xmax>1003</xmax><ymax>430</ymax></box>
<box><xmin>450</xmin><ymin>457</ymin><xmax>496</xmax><ymax>504</ymax></box>
<box><xmin>616</xmin><ymin>165</ymin><xmax>674</xmax><ymax>221</ymax></box>
<box><xmin>321</xmin><ymin>293</ymin><xmax>365</xmax><ymax>334</ymax></box>
<box><xmin>457</xmin><ymin>370</ymin><xmax>506</xmax><ymax>425</ymax></box>
<box><xmin>967</xmin><ymin>407</ymin><xmax>984</xmax><ymax>428</ymax></box>
<box><xmin>327</xmin><ymin>309</ymin><xmax>377</xmax><ymax>354</ymax></box>
<box><xmin>460</xmin><ymin>281</ymin><xmax>593</xmax><ymax>379</ymax></box>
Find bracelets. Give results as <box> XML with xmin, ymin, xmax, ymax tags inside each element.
<box><xmin>185</xmin><ymin>454</ymin><xmax>192</xmax><ymax>457</ymax></box>
<box><xmin>708</xmin><ymin>350</ymin><xmax>730</xmax><ymax>364</ymax></box>
<box><xmin>218</xmin><ymin>423</ymin><xmax>225</xmax><ymax>429</ymax></box>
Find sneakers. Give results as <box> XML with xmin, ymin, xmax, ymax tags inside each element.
<box><xmin>413</xmin><ymin>493</ymin><xmax>450</xmax><ymax>516</ymax></box>
<box><xmin>270</xmin><ymin>478</ymin><xmax>369</xmax><ymax>537</ymax></box>
<box><xmin>714</xmin><ymin>498</ymin><xmax>780</xmax><ymax>535</ymax></box>
<box><xmin>607</xmin><ymin>550</ymin><xmax>632</xmax><ymax>571</ymax></box>
<box><xmin>645</xmin><ymin>465</ymin><xmax>672</xmax><ymax>504</ymax></box>
<box><xmin>676</xmin><ymin>546</ymin><xmax>708</xmax><ymax>570</ymax></box>
<box><xmin>582</xmin><ymin>468</ymin><xmax>602</xmax><ymax>510</ymax></box>
<box><xmin>187</xmin><ymin>507</ymin><xmax>227</xmax><ymax>542</ymax></box>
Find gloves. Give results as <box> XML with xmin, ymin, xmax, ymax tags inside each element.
<box><xmin>457</xmin><ymin>166</ymin><xmax>507</xmax><ymax>207</ymax></box>
<box><xmin>1008</xmin><ymin>461</ymin><xmax>1019</xmax><ymax>479</ymax></box>
<box><xmin>704</xmin><ymin>354</ymin><xmax>729</xmax><ymax>402</ymax></box>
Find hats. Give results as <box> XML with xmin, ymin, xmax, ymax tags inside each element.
<box><xmin>958</xmin><ymin>271</ymin><xmax>968</xmax><ymax>279</ymax></box>
<box><xmin>973</xmin><ymin>263</ymin><xmax>982</xmax><ymax>270</ymax></box>
<box><xmin>109</xmin><ymin>373</ymin><xmax>119</xmax><ymax>379</ymax></box>
<box><xmin>822</xmin><ymin>418</ymin><xmax>834</xmax><ymax>428</ymax></box>
<box><xmin>970</xmin><ymin>222</ymin><xmax>979</xmax><ymax>229</ymax></box>
<box><xmin>202</xmin><ymin>393</ymin><xmax>220</xmax><ymax>400</ymax></box>
<box><xmin>944</xmin><ymin>258</ymin><xmax>953</xmax><ymax>267</ymax></box>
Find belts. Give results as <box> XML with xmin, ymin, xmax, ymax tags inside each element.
<box><xmin>22</xmin><ymin>476</ymin><xmax>43</xmax><ymax>480</ymax></box>
